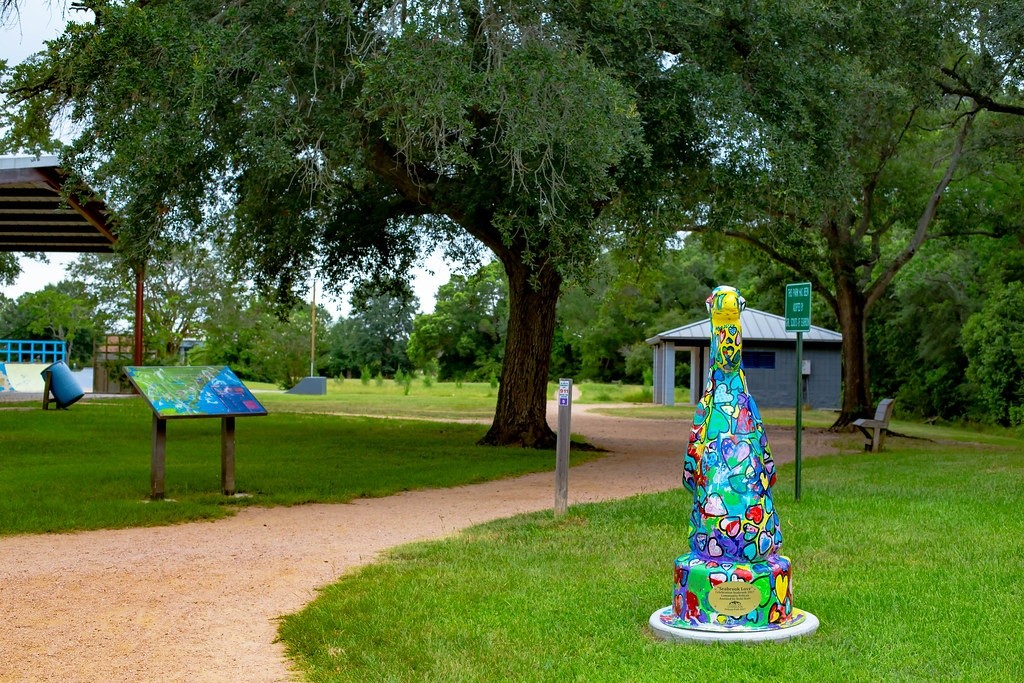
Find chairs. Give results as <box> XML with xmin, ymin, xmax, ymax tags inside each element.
<box><xmin>853</xmin><ymin>398</ymin><xmax>896</xmax><ymax>451</ymax></box>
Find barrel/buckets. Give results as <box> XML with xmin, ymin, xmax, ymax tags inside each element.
<box><xmin>40</xmin><ymin>360</ymin><xmax>86</xmax><ymax>407</ymax></box>
